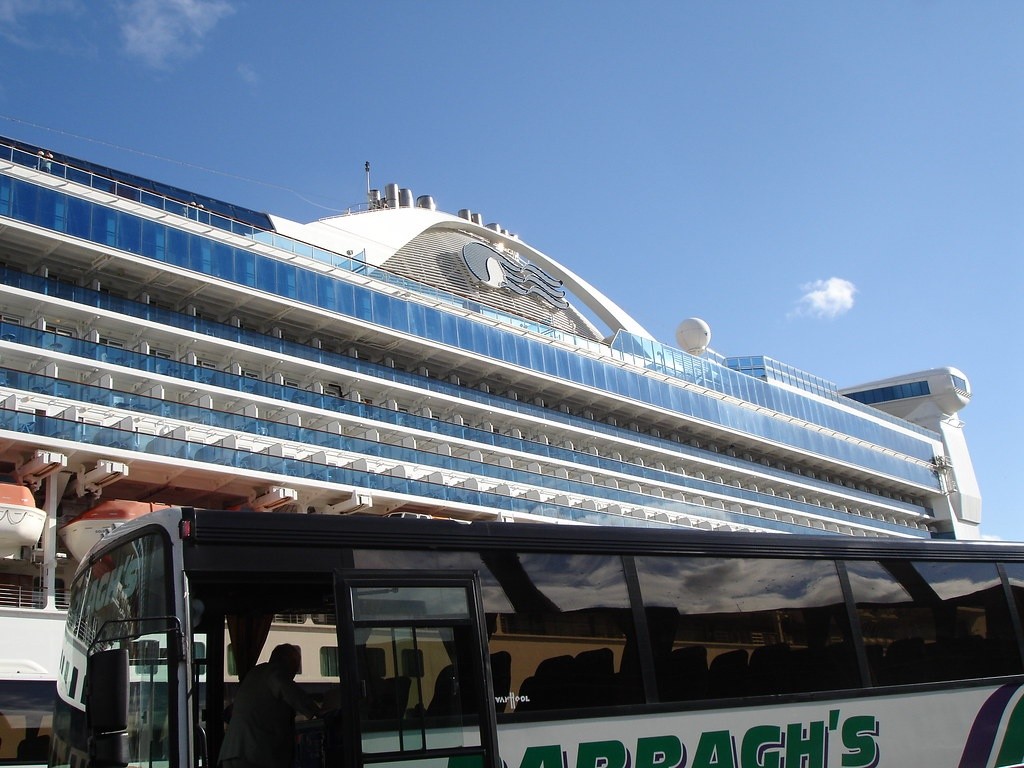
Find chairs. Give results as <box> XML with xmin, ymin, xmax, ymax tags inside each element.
<box><xmin>361</xmin><ymin>635</ymin><xmax>993</xmax><ymax>719</ymax></box>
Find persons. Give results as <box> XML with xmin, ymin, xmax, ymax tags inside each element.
<box><xmin>216</xmin><ymin>644</ymin><xmax>336</xmax><ymax>768</ymax></box>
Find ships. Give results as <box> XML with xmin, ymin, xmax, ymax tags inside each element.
<box><xmin>0</xmin><ymin>130</ymin><xmax>1003</xmax><ymax>768</ymax></box>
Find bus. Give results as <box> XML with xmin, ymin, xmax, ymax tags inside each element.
<box><xmin>45</xmin><ymin>508</ymin><xmax>1023</xmax><ymax>768</ymax></box>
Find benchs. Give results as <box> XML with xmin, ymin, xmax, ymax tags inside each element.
<box><xmin>17</xmin><ymin>735</ymin><xmax>50</xmax><ymax>760</ymax></box>
